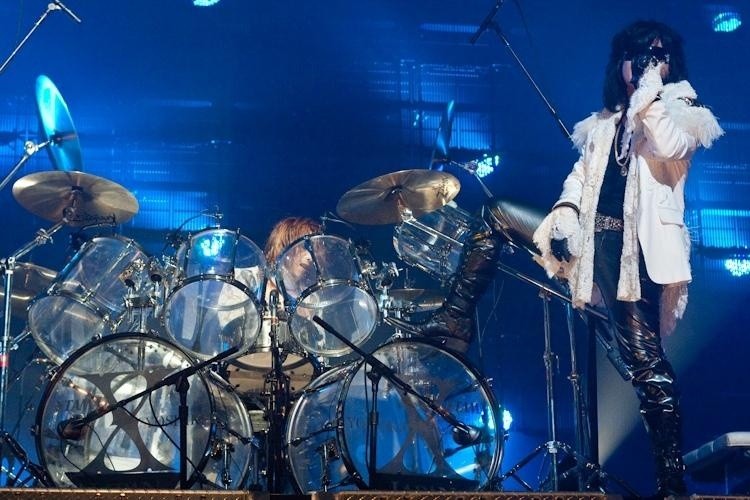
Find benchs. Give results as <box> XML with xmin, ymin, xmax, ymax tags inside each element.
<box><xmin>682</xmin><ymin>431</ymin><xmax>750</xmax><ymax>496</ymax></box>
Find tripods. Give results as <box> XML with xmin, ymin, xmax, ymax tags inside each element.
<box><xmin>0</xmin><ymin>189</ymin><xmax>118</xmax><ymax>488</ymax></box>
<box><xmin>477</xmin><ymin>274</ymin><xmax>645</xmax><ymax>500</ymax></box>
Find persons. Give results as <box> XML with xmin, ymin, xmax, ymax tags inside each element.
<box><xmin>530</xmin><ymin>18</ymin><xmax>730</xmax><ymax>499</ymax></box>
<box><xmin>214</xmin><ymin>214</ymin><xmax>332</xmax><ymax>359</ymax></box>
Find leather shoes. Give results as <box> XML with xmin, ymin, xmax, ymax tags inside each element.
<box><xmin>382</xmin><ymin>307</ymin><xmax>477</xmax><ymax>363</ymax></box>
<box><xmin>656</xmin><ymin>472</ymin><xmax>690</xmax><ymax>500</ymax></box>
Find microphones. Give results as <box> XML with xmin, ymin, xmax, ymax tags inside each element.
<box><xmin>55</xmin><ymin>0</ymin><xmax>80</xmax><ymax>25</ymax></box>
<box><xmin>631</xmin><ymin>53</ymin><xmax>658</xmax><ymax>78</ymax></box>
<box><xmin>469</xmin><ymin>0</ymin><xmax>505</xmax><ymax>46</ymax></box>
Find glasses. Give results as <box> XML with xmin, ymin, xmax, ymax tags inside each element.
<box><xmin>623</xmin><ymin>45</ymin><xmax>667</xmax><ymax>60</ymax></box>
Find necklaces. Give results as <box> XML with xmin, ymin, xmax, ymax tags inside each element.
<box><xmin>613</xmin><ymin>119</ymin><xmax>636</xmax><ymax>178</ymax></box>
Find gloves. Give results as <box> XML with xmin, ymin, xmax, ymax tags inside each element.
<box><xmin>629</xmin><ymin>46</ymin><xmax>661</xmax><ymax>91</ymax></box>
<box><xmin>549</xmin><ymin>236</ymin><xmax>573</xmax><ymax>263</ymax></box>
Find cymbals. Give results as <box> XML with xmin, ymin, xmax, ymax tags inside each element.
<box><xmin>336</xmin><ymin>169</ymin><xmax>460</xmax><ymax>226</ymax></box>
<box><xmin>374</xmin><ymin>288</ymin><xmax>443</xmax><ymax>314</ymax></box>
<box><xmin>13</xmin><ymin>171</ymin><xmax>140</xmax><ymax>226</ymax></box>
<box><xmin>35</xmin><ymin>75</ymin><xmax>83</xmax><ymax>172</ymax></box>
<box><xmin>0</xmin><ymin>261</ymin><xmax>58</xmax><ymax>300</ymax></box>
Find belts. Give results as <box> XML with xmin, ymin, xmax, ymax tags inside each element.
<box><xmin>594</xmin><ymin>214</ymin><xmax>624</xmax><ymax>232</ymax></box>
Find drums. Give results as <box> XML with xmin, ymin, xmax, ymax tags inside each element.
<box><xmin>285</xmin><ymin>336</ymin><xmax>505</xmax><ymax>493</ymax></box>
<box><xmin>222</xmin><ymin>309</ymin><xmax>323</xmax><ymax>400</ymax></box>
<box><xmin>29</xmin><ymin>235</ymin><xmax>165</xmax><ymax>375</ymax></box>
<box><xmin>166</xmin><ymin>228</ymin><xmax>266</xmax><ymax>362</ymax></box>
<box><xmin>34</xmin><ymin>333</ymin><xmax>254</xmax><ymax>490</ymax></box>
<box><xmin>275</xmin><ymin>233</ymin><xmax>379</xmax><ymax>357</ymax></box>
<box><xmin>393</xmin><ymin>200</ymin><xmax>500</xmax><ymax>302</ymax></box>
